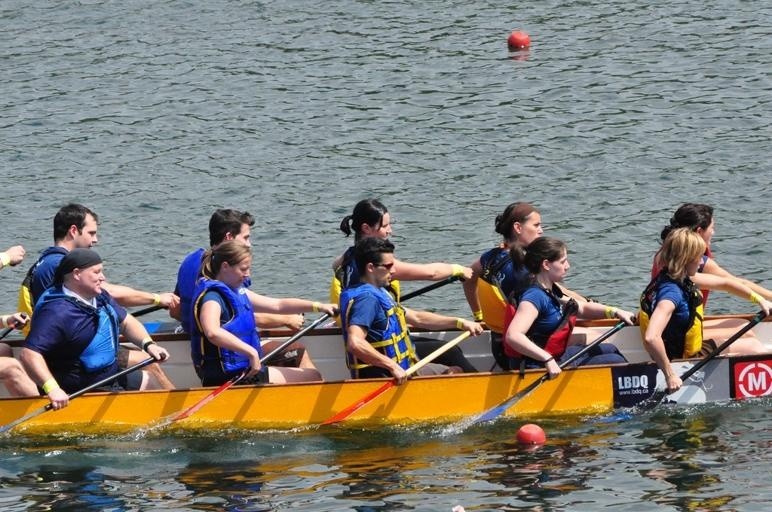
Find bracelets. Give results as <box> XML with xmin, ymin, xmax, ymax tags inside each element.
<box><xmin>152</xmin><ymin>293</ymin><xmax>160</xmax><ymax>305</ymax></box>
<box><xmin>543</xmin><ymin>357</ymin><xmax>554</xmax><ymax>363</ymax></box>
<box><xmin>312</xmin><ymin>301</ymin><xmax>320</xmax><ymax>312</ymax></box>
<box><xmin>452</xmin><ymin>263</ymin><xmax>465</xmax><ymax>277</ymax></box>
<box><xmin>750</xmin><ymin>291</ymin><xmax>764</xmax><ymax>305</ymax></box>
<box><xmin>141</xmin><ymin>336</ymin><xmax>155</xmax><ymax>352</ymax></box>
<box><xmin>455</xmin><ymin>317</ymin><xmax>465</xmax><ymax>329</ymax></box>
<box><xmin>41</xmin><ymin>378</ymin><xmax>60</xmax><ymax>393</ymax></box>
<box><xmin>0</xmin><ymin>251</ymin><xmax>12</xmax><ymax>266</ymax></box>
<box><xmin>2</xmin><ymin>315</ymin><xmax>11</xmax><ymax>329</ymax></box>
<box><xmin>604</xmin><ymin>305</ymin><xmax>618</xmax><ymax>319</ymax></box>
<box><xmin>472</xmin><ymin>310</ymin><xmax>483</xmax><ymax>321</ymax></box>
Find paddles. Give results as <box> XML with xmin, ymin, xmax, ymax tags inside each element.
<box><xmin>171</xmin><ymin>304</ymin><xmax>340</xmax><ymax>422</ymax></box>
<box><xmin>2</xmin><ymin>354</ymin><xmax>168</xmax><ymax>433</ymax></box>
<box><xmin>320</xmin><ymin>320</ymin><xmax>490</xmax><ymax>428</ymax></box>
<box><xmin>642</xmin><ymin>306</ymin><xmax>772</xmax><ymax>412</ymax></box>
<box><xmin>471</xmin><ymin>314</ymin><xmax>640</xmax><ymax>425</ymax></box>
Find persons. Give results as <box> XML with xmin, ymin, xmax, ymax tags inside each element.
<box><xmin>332</xmin><ymin>197</ymin><xmax>479</xmax><ymax>373</ymax></box>
<box><xmin>648</xmin><ymin>201</ymin><xmax>771</xmax><ymax>340</ymax></box>
<box><xmin>18</xmin><ymin>203</ymin><xmax>182</xmax><ymax>390</ymax></box>
<box><xmin>339</xmin><ymin>235</ymin><xmax>483</xmax><ymax>387</ymax></box>
<box><xmin>170</xmin><ymin>208</ymin><xmax>339</xmax><ymax>370</ymax></box>
<box><xmin>18</xmin><ymin>246</ymin><xmax>171</xmax><ymax>413</ymax></box>
<box><xmin>189</xmin><ymin>239</ymin><xmax>322</xmax><ymax>387</ymax></box>
<box><xmin>462</xmin><ymin>202</ymin><xmax>630</xmax><ymax>366</ymax></box>
<box><xmin>500</xmin><ymin>237</ymin><xmax>638</xmax><ymax>380</ymax></box>
<box><xmin>0</xmin><ymin>313</ymin><xmax>40</xmax><ymax>399</ymax></box>
<box><xmin>0</xmin><ymin>244</ymin><xmax>26</xmax><ymax>356</ymax></box>
<box><xmin>640</xmin><ymin>226</ymin><xmax>772</xmax><ymax>394</ymax></box>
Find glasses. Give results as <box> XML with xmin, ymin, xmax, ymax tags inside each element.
<box><xmin>374</xmin><ymin>263</ymin><xmax>393</xmax><ymax>269</ymax></box>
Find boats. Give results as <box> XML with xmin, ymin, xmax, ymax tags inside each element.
<box><xmin>0</xmin><ymin>313</ymin><xmax>772</xmax><ymax>428</ymax></box>
